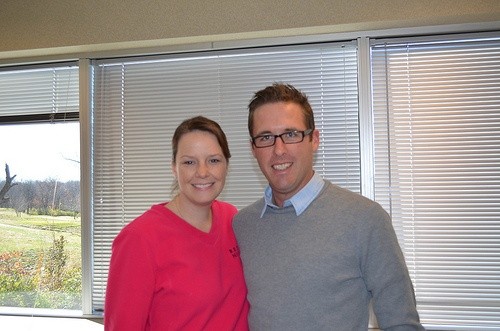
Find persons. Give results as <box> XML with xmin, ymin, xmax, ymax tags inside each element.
<box><xmin>103</xmin><ymin>115</ymin><xmax>251</xmax><ymax>330</ymax></box>
<box><xmin>231</xmin><ymin>83</ymin><xmax>425</xmax><ymax>330</ymax></box>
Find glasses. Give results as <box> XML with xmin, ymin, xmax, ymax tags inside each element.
<box><xmin>252</xmin><ymin>128</ymin><xmax>314</xmax><ymax>148</ymax></box>
